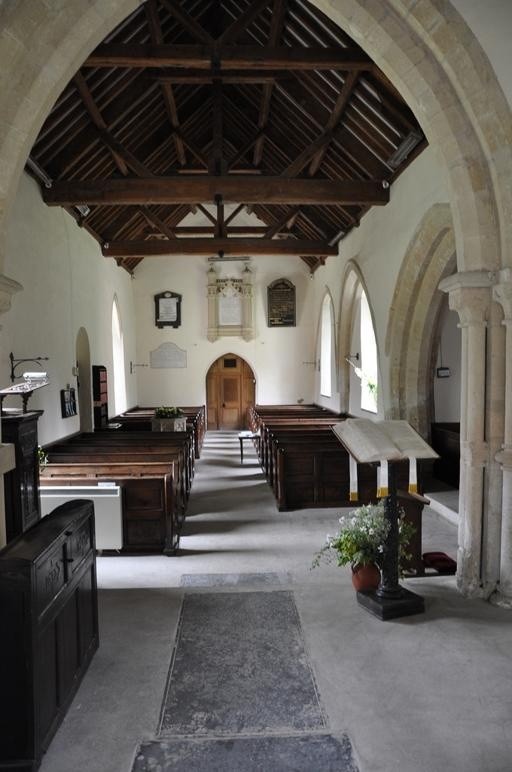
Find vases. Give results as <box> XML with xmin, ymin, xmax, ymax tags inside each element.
<box><xmin>351</xmin><ymin>561</ymin><xmax>380</xmax><ymax>595</ymax></box>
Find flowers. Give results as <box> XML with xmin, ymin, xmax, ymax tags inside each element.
<box><xmin>308</xmin><ymin>501</ymin><xmax>417</xmax><ymax>580</ymax></box>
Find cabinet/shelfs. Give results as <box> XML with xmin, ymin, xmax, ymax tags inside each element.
<box><xmin>0</xmin><ymin>498</ymin><xmax>100</xmax><ymax>772</ymax></box>
